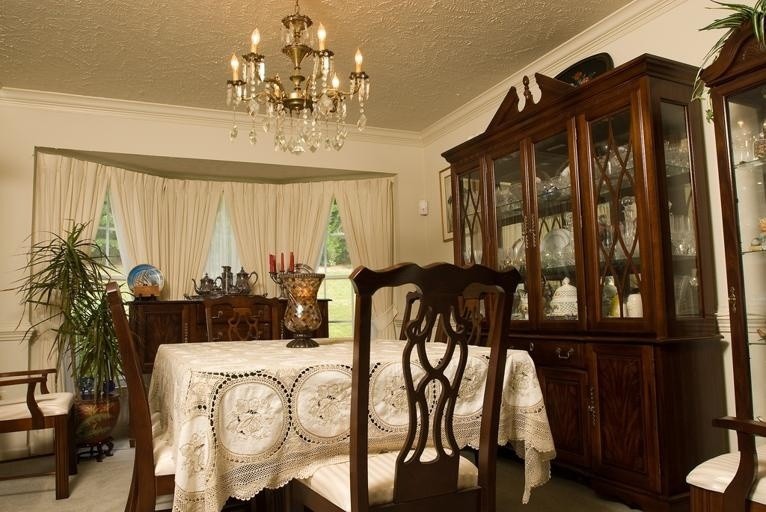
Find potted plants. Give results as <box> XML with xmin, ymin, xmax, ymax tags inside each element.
<box><xmin>4</xmin><ymin>216</ymin><xmax>122</xmax><ymax>467</ymax></box>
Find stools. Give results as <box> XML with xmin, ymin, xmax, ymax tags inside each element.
<box><xmin>685</xmin><ymin>450</ymin><xmax>766</xmax><ymax>512</ymax></box>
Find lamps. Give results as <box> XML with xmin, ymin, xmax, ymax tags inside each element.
<box><xmin>225</xmin><ymin>0</ymin><xmax>370</xmax><ymax>155</ymax></box>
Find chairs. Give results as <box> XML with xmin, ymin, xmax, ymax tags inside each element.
<box><xmin>205</xmin><ymin>295</ymin><xmax>274</xmax><ymax>341</ymax></box>
<box><xmin>104</xmin><ymin>281</ymin><xmax>175</xmax><ymax>512</ymax></box>
<box><xmin>0</xmin><ymin>368</ymin><xmax>79</xmax><ymax>500</ymax></box>
<box><xmin>284</xmin><ymin>265</ymin><xmax>524</xmax><ymax>512</ymax></box>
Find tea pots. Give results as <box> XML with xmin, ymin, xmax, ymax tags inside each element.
<box><xmin>228</xmin><ymin>267</ymin><xmax>258</xmax><ymax>292</ymax></box>
<box><xmin>192</xmin><ymin>274</ymin><xmax>222</xmax><ymax>292</ymax></box>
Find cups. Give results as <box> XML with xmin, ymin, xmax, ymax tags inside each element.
<box><xmin>502</xmin><ymin>213</ymin><xmax>698</xmax><ymax>273</ymax></box>
<box><xmin>499</xmin><ymin>137</ymin><xmax>688</xmax><ymax>209</ymax></box>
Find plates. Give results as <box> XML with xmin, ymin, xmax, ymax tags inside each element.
<box><xmin>287</xmin><ymin>264</ymin><xmax>316</xmax><ymax>274</ymax></box>
<box><xmin>128</xmin><ymin>264</ymin><xmax>164</xmax><ymax>295</ymax></box>
<box><xmin>538</xmin><ymin>229</ymin><xmax>571</xmax><ymax>264</ymax></box>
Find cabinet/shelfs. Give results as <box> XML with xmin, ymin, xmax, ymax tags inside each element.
<box><xmin>585</xmin><ymin>337</ymin><xmax>723</xmax><ymax>498</ymax></box>
<box><xmin>484</xmin><ymin>113</ymin><xmax>587</xmax><ymax>327</ymax></box>
<box><xmin>123</xmin><ymin>300</ymin><xmax>332</xmax><ymax>375</ymax></box>
<box><xmin>699</xmin><ymin>15</ymin><xmax>766</xmax><ymax>451</ymax></box>
<box><xmin>520</xmin><ymin>338</ymin><xmax>587</xmax><ymax>478</ymax></box>
<box><xmin>574</xmin><ymin>76</ymin><xmax>721</xmax><ymax>335</ymax></box>
<box><xmin>449</xmin><ymin>146</ymin><xmax>490</xmax><ymax>269</ymax></box>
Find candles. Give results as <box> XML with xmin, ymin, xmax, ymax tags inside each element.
<box><xmin>280</xmin><ymin>252</ymin><xmax>283</xmax><ymax>272</ymax></box>
<box><xmin>269</xmin><ymin>254</ymin><xmax>276</xmax><ymax>273</ymax></box>
<box><xmin>289</xmin><ymin>251</ymin><xmax>293</xmax><ymax>271</ymax></box>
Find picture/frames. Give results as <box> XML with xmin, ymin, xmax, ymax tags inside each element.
<box><xmin>438</xmin><ymin>165</ymin><xmax>478</xmax><ymax>243</ymax></box>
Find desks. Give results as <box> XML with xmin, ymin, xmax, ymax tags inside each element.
<box><xmin>149</xmin><ymin>338</ymin><xmax>556</xmax><ymax>512</ymax></box>
